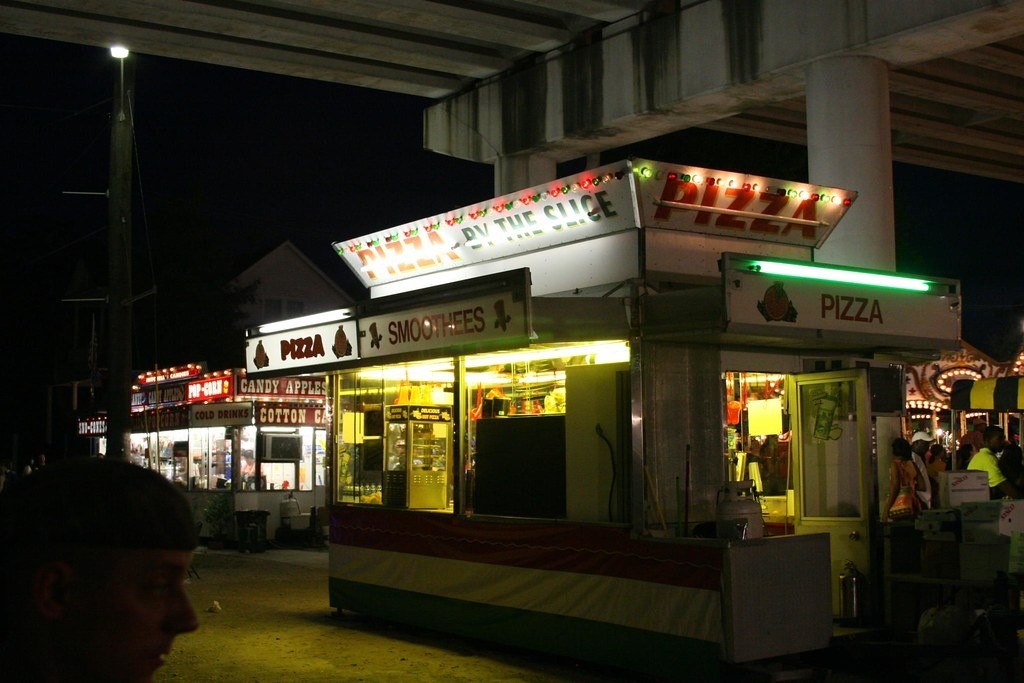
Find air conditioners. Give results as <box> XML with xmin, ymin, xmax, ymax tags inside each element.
<box><xmin>260</xmin><ymin>434</ymin><xmax>302</xmax><ymax>461</ymax></box>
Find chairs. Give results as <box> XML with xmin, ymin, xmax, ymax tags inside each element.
<box><xmin>186</xmin><ymin>521</ymin><xmax>203</xmax><ymax>579</ymax></box>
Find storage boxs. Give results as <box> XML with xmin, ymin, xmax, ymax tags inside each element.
<box><xmin>915</xmin><ymin>469</ymin><xmax>1024</xmax><ymax>581</ymax></box>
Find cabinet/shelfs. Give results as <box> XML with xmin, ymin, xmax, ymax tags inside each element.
<box><xmin>383</xmin><ymin>405</ymin><xmax>452</xmax><ymax>509</ymax></box>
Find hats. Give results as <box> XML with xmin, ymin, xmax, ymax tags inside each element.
<box><xmin>912</xmin><ymin>431</ymin><xmax>934</xmax><ymax>442</ymax></box>
<box><xmin>0</xmin><ymin>458</ymin><xmax>199</xmax><ymax>551</ymax></box>
<box><xmin>973</xmin><ymin>419</ymin><xmax>986</xmax><ymax>425</ymax></box>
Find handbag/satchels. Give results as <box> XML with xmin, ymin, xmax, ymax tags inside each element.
<box><xmin>883</xmin><ymin>459</ymin><xmax>913</xmax><ymax>519</ymax></box>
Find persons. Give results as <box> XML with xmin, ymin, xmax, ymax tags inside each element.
<box><xmin>882</xmin><ymin>437</ymin><xmax>927</xmax><ymax>519</ymax></box>
<box><xmin>223</xmin><ymin>448</ymin><xmax>264</xmax><ymax>482</ymax></box>
<box><xmin>966</xmin><ymin>424</ymin><xmax>1024</xmax><ymax>499</ymax></box>
<box><xmin>0</xmin><ymin>456</ymin><xmax>200</xmax><ymax>683</ymax></box>
<box><xmin>927</xmin><ymin>445</ymin><xmax>946</xmax><ymax>497</ymax></box>
<box><xmin>0</xmin><ymin>454</ymin><xmax>48</xmax><ymax>494</ymax></box>
<box><xmin>961</xmin><ymin>444</ymin><xmax>977</xmax><ymax>467</ymax></box>
<box><xmin>910</xmin><ymin>431</ymin><xmax>937</xmax><ymax>509</ymax></box>
<box><xmin>997</xmin><ymin>444</ymin><xmax>1024</xmax><ymax>487</ymax></box>
<box><xmin>960</xmin><ymin>418</ymin><xmax>988</xmax><ymax>449</ymax></box>
